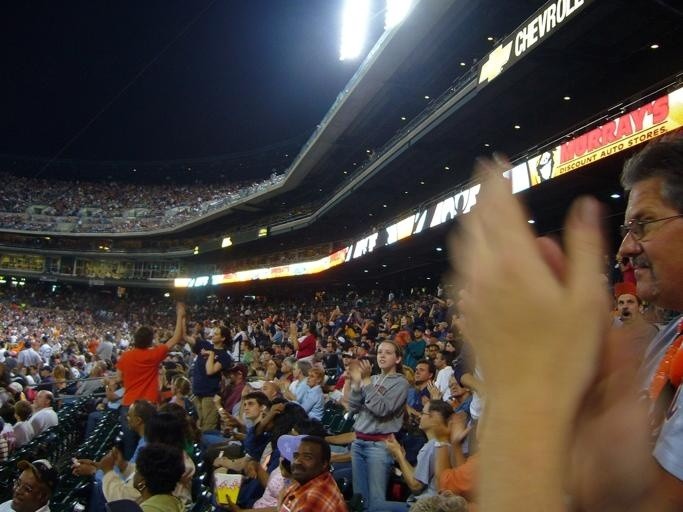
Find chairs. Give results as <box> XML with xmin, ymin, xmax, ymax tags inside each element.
<box><xmin>0</xmin><ymin>374</ymin><xmax>421</xmax><ymax>511</ymax></box>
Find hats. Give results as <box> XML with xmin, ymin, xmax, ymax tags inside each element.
<box><xmin>16</xmin><ymin>458</ymin><xmax>60</xmax><ymax>495</ymax></box>
<box><xmin>614</xmin><ymin>281</ymin><xmax>642</xmax><ymax>302</ymax></box>
<box><xmin>277</xmin><ymin>433</ymin><xmax>310</xmax><ymax>461</ymax></box>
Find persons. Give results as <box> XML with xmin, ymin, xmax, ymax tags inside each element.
<box><xmin>620</xmin><ymin>135</ymin><xmax>683</xmax><ymax>510</ymax></box>
<box><xmin>451</xmin><ymin>157</ymin><xmax>683</xmax><ymax>510</ymax></box>
<box><xmin>1</xmin><ymin>171</ymin><xmax>644</xmax><ymax>511</ymax></box>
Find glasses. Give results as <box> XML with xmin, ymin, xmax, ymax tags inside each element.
<box><xmin>11</xmin><ymin>477</ymin><xmax>34</xmax><ymax>493</ymax></box>
<box><xmin>620</xmin><ymin>215</ymin><xmax>683</xmax><ymax>240</ymax></box>
